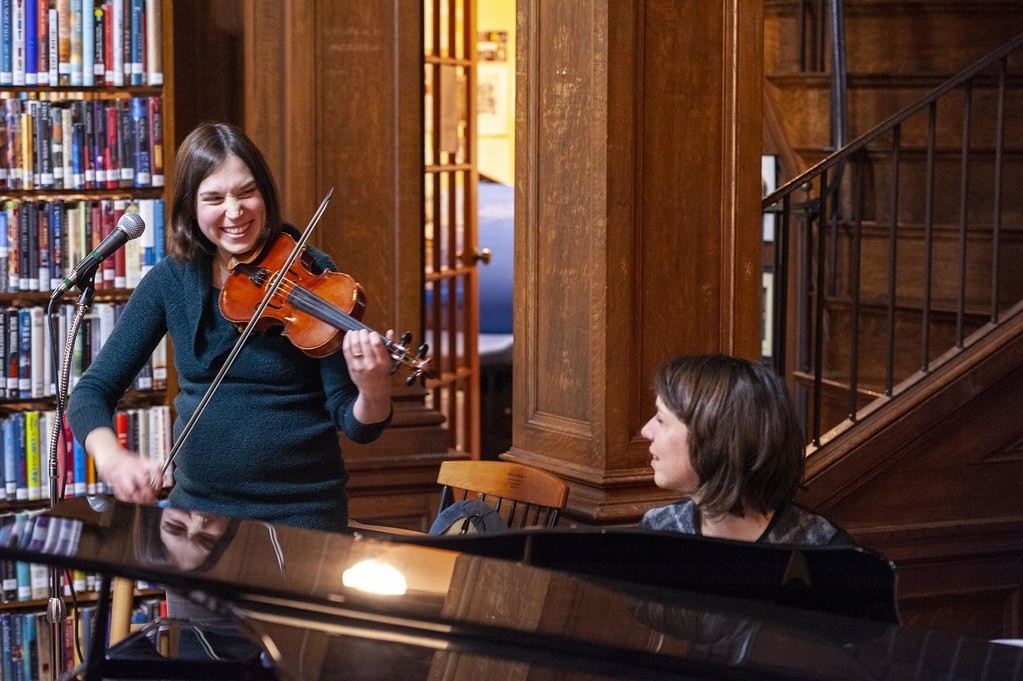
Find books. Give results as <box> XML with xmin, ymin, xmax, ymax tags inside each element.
<box><xmin>0</xmin><ymin>513</ymin><xmax>83</xmax><ymax>558</ymax></box>
<box><xmin>0</xmin><ymin>0</ymin><xmax>174</xmax><ymax>681</ymax></box>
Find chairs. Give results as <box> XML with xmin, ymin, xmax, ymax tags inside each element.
<box><xmin>437</xmin><ymin>460</ymin><xmax>569</xmax><ymax>533</ymax></box>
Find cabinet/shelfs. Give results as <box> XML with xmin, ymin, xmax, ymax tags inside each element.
<box><xmin>0</xmin><ymin>0</ymin><xmax>180</xmax><ymax>681</ymax></box>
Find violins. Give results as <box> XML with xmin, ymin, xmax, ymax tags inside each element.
<box><xmin>218</xmin><ymin>230</ymin><xmax>434</xmax><ymax>385</ymax></box>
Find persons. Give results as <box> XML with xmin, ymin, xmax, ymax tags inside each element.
<box><xmin>641</xmin><ymin>355</ymin><xmax>856</xmax><ymax>551</ymax></box>
<box><xmin>66</xmin><ymin>124</ymin><xmax>395</xmax><ymax>536</ymax></box>
<box><xmin>135</xmin><ymin>505</ymin><xmax>241</xmax><ymax>577</ymax></box>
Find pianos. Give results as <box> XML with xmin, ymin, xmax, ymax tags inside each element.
<box><xmin>0</xmin><ymin>489</ymin><xmax>1023</xmax><ymax>681</ymax></box>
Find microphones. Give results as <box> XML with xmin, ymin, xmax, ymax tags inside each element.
<box><xmin>86</xmin><ymin>496</ymin><xmax>111</xmax><ymax>513</ymax></box>
<box><xmin>50</xmin><ymin>212</ymin><xmax>146</xmax><ymax>301</ymax></box>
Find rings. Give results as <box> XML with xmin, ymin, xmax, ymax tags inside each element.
<box><xmin>352</xmin><ymin>351</ymin><xmax>365</xmax><ymax>358</ymax></box>
